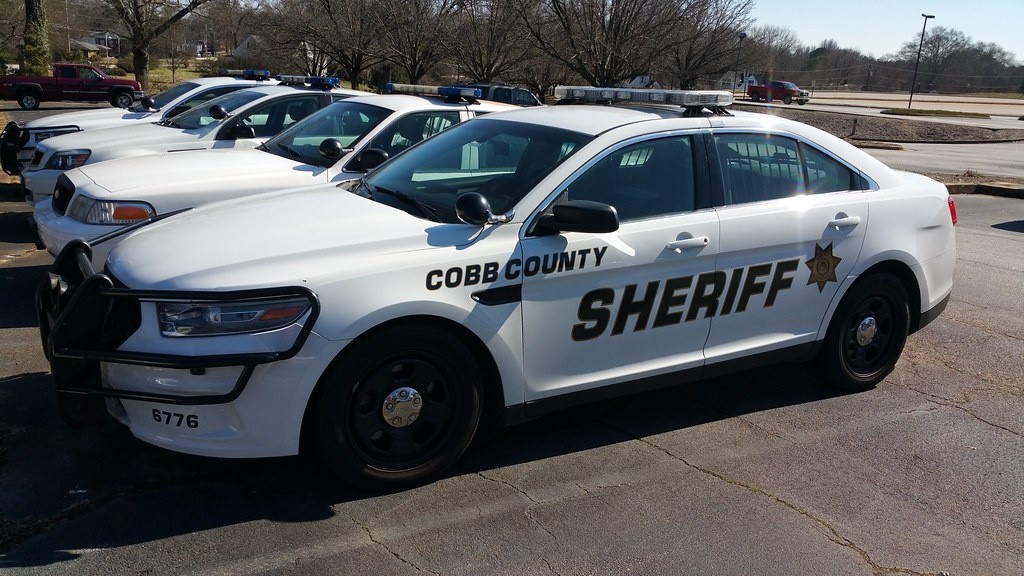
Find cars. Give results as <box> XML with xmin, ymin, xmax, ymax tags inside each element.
<box><xmin>1</xmin><ymin>70</ymin><xmax>378</xmax><ymax>204</ymax></box>
<box><xmin>34</xmin><ymin>83</ymin><xmax>530</xmax><ymax>253</ymax></box>
<box><xmin>38</xmin><ymin>84</ymin><xmax>956</xmax><ymax>481</ymax></box>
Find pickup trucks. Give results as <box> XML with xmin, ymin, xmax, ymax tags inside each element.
<box><xmin>0</xmin><ymin>61</ymin><xmax>143</xmax><ymax>109</ymax></box>
<box><xmin>747</xmin><ymin>80</ymin><xmax>811</xmax><ymax>105</ymax></box>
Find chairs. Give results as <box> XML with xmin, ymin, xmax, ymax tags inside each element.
<box><xmin>287</xmin><ymin>105</ymin><xmax>305</xmax><ymax>128</ymax></box>
<box><xmin>387</xmin><ymin>119</ymin><xmax>424</xmax><ymax>158</ymax></box>
<box><xmin>615</xmin><ymin>141</ymin><xmax>694</xmax><ymax>217</ymax></box>
